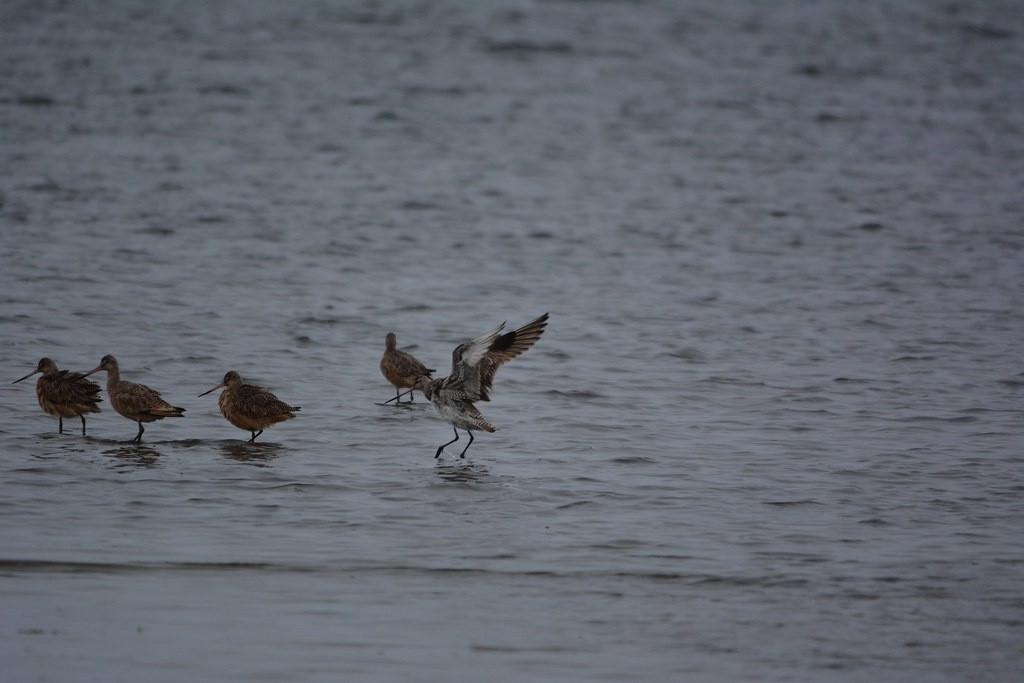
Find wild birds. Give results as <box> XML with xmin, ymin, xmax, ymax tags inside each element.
<box><xmin>13</xmin><ymin>356</ymin><xmax>102</xmax><ymax>437</ymax></box>
<box><xmin>197</xmin><ymin>370</ymin><xmax>302</xmax><ymax>445</ymax></box>
<box><xmin>378</xmin><ymin>332</ymin><xmax>438</xmax><ymax>404</ymax></box>
<box><xmin>382</xmin><ymin>312</ymin><xmax>549</xmax><ymax>459</ymax></box>
<box><xmin>71</xmin><ymin>356</ymin><xmax>185</xmax><ymax>445</ymax></box>
